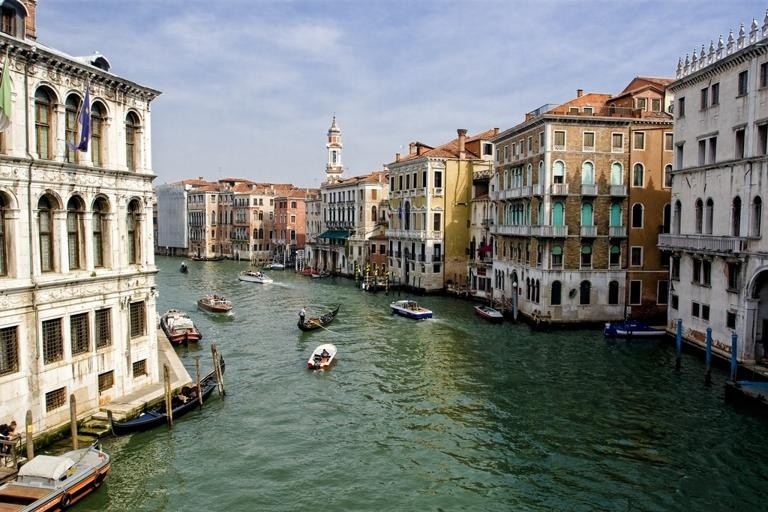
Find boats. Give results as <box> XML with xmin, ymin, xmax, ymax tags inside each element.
<box><xmin>159</xmin><ymin>307</ymin><xmax>202</xmax><ymax>345</ymax></box>
<box><xmin>474</xmin><ymin>303</ymin><xmax>505</xmax><ymax>323</ymax></box>
<box><xmin>388</xmin><ymin>300</ymin><xmax>433</xmax><ymax>321</ymax></box>
<box><xmin>302</xmin><ymin>266</ymin><xmax>330</xmax><ymax>278</ymax></box>
<box><xmin>0</xmin><ymin>447</ymin><xmax>113</xmax><ymax>512</ymax></box>
<box><xmin>238</xmin><ymin>269</ymin><xmax>273</xmax><ymax>285</ymax></box>
<box><xmin>178</xmin><ymin>264</ymin><xmax>189</xmax><ymax>273</ymax></box>
<box><xmin>196</xmin><ymin>295</ymin><xmax>233</xmax><ymax>314</ymax></box>
<box><xmin>601</xmin><ymin>317</ymin><xmax>667</xmax><ymax>341</ymax></box>
<box><xmin>296</xmin><ymin>302</ymin><xmax>343</xmax><ymax>332</ymax></box>
<box><xmin>261</xmin><ymin>262</ymin><xmax>285</xmax><ymax>270</ymax></box>
<box><xmin>104</xmin><ymin>353</ymin><xmax>227</xmax><ymax>438</ymax></box>
<box><xmin>305</xmin><ymin>343</ymin><xmax>338</xmax><ymax>371</ymax></box>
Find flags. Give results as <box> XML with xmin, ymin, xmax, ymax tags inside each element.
<box><xmin>0</xmin><ymin>46</ymin><xmax>11</xmax><ymax>118</ymax></box>
<box><xmin>67</xmin><ymin>81</ymin><xmax>90</xmax><ymax>152</ymax></box>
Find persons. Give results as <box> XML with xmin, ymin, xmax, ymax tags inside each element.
<box><xmin>320</xmin><ymin>349</ymin><xmax>329</xmax><ymax>364</ymax></box>
<box><xmin>177</xmin><ymin>384</ymin><xmax>198</xmax><ymax>404</ymax></box>
<box><xmin>0</xmin><ymin>421</ymin><xmax>16</xmax><ymax>462</ymax></box>
<box><xmin>299</xmin><ymin>306</ymin><xmax>307</xmax><ymax>326</ymax></box>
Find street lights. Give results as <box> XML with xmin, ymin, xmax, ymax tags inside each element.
<box><xmin>511</xmin><ymin>281</ymin><xmax>517</xmax><ymax>306</ymax></box>
